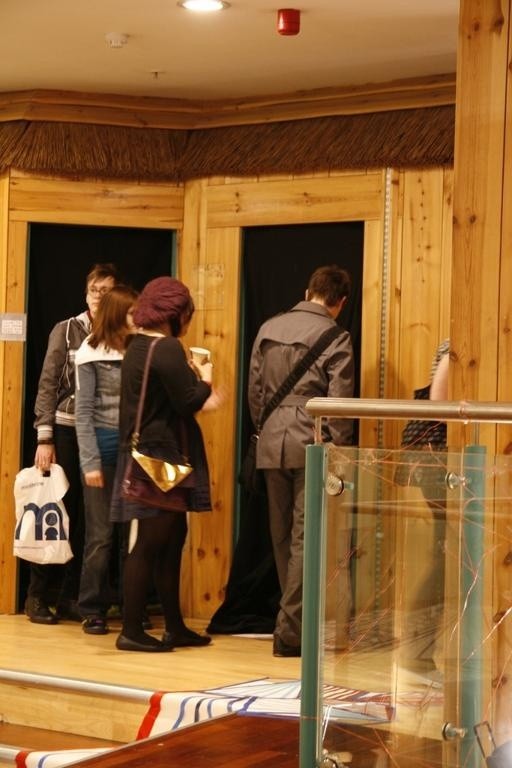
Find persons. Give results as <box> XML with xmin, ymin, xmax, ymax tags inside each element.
<box><xmin>21</xmin><ymin>263</ymin><xmax>214</xmax><ymax>652</ymax></box>
<box><xmin>391</xmin><ymin>337</ymin><xmax>450</xmax><ymax>690</ymax></box>
<box><xmin>248</xmin><ymin>265</ymin><xmax>355</xmax><ymax>657</ymax></box>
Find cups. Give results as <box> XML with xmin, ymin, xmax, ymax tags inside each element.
<box><xmin>189</xmin><ymin>347</ymin><xmax>210</xmax><ymax>369</ymax></box>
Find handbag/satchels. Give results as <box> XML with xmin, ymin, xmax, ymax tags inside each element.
<box><xmin>241</xmin><ymin>434</ymin><xmax>268</xmax><ymax>508</ymax></box>
<box><xmin>12</xmin><ymin>463</ymin><xmax>74</xmax><ymax>566</ymax></box>
<box><xmin>120</xmin><ymin>449</ymin><xmax>195</xmax><ymax>512</ymax></box>
<box><xmin>393</xmin><ymin>384</ymin><xmax>447</xmax><ymax>486</ymax></box>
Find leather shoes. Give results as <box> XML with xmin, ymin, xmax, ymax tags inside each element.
<box><xmin>274</xmin><ymin>636</ymin><xmax>300</xmax><ymax>658</ymax></box>
<box><xmin>25</xmin><ymin>591</ymin><xmax>210</xmax><ymax>651</ymax></box>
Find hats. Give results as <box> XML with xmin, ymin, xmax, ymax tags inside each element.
<box><xmin>133</xmin><ymin>276</ymin><xmax>190</xmax><ymax>327</ymax></box>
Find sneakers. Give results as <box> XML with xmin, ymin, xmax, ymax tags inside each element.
<box><xmin>392</xmin><ymin>660</ymin><xmax>443</xmax><ymax>690</ymax></box>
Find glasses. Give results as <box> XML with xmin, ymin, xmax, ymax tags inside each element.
<box><xmin>84</xmin><ymin>286</ymin><xmax>111</xmax><ymax>297</ymax></box>
